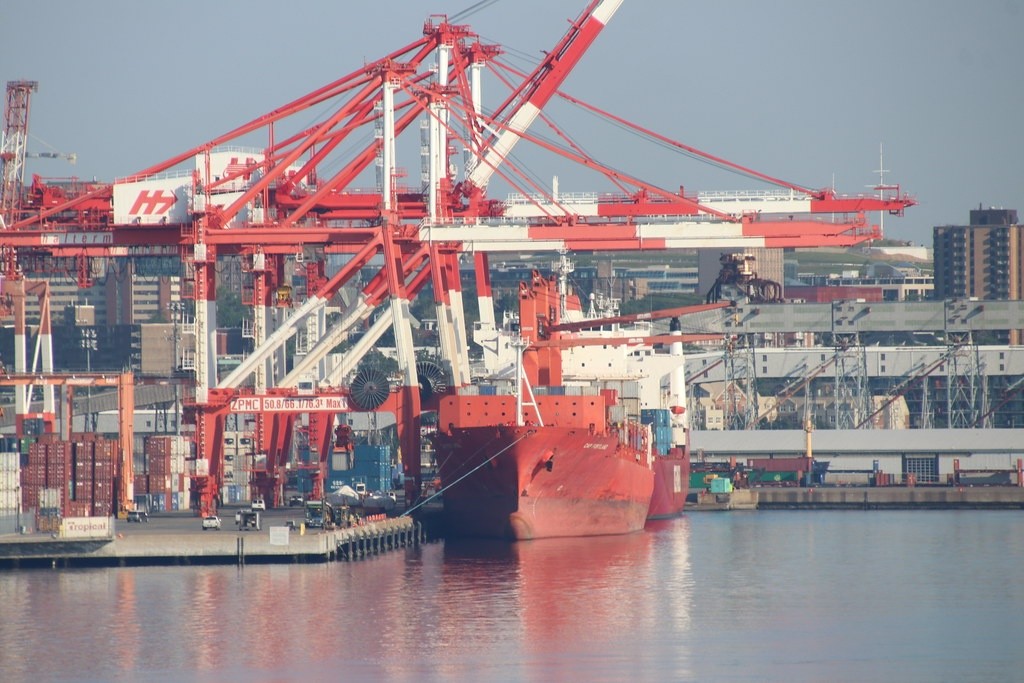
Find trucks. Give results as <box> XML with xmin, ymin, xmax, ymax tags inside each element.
<box><xmin>239</xmin><ymin>511</ymin><xmax>262</xmax><ymax>532</ymax></box>
<box><xmin>304</xmin><ymin>501</ymin><xmax>322</xmax><ymax>528</ymax></box>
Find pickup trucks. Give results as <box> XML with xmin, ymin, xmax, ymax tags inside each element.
<box><xmin>202</xmin><ymin>515</ymin><xmax>222</xmax><ymax>530</ymax></box>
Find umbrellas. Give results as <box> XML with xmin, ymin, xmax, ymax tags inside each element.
<box><xmin>333</xmin><ymin>484</ymin><xmax>359</xmax><ymax>507</ymax></box>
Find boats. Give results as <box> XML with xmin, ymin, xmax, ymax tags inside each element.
<box><xmin>474</xmin><ymin>249</ymin><xmax>699</xmax><ymax>520</ymax></box>
<box><xmin>430</xmin><ymin>264</ymin><xmax>740</xmax><ymax>543</ymax></box>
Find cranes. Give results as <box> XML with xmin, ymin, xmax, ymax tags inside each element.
<box><xmin>26</xmin><ymin>131</ymin><xmax>77</xmax><ymax>165</ymax></box>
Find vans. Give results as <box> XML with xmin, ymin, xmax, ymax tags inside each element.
<box><xmin>252</xmin><ymin>498</ymin><xmax>266</xmax><ymax>512</ymax></box>
<box><xmin>235</xmin><ymin>510</ymin><xmax>249</xmax><ymax>524</ymax></box>
<box><xmin>127</xmin><ymin>511</ymin><xmax>149</xmax><ymax>523</ymax></box>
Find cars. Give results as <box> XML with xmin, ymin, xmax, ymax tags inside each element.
<box><xmin>354</xmin><ymin>482</ymin><xmax>398</xmax><ymax>504</ymax></box>
